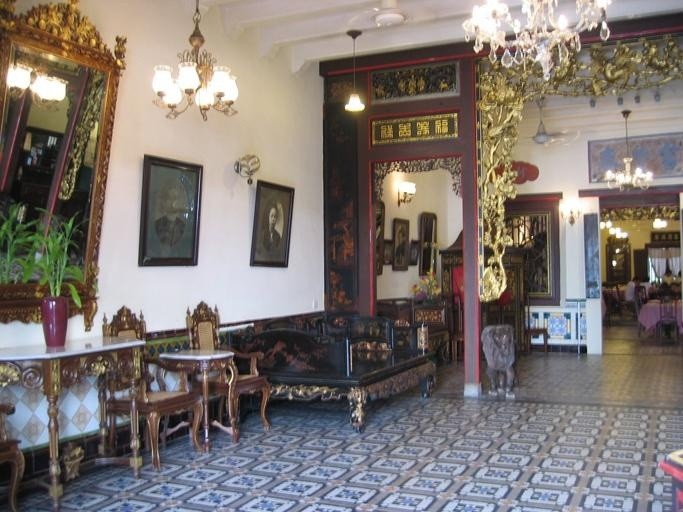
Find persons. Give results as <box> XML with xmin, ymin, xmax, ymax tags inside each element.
<box><xmin>395</xmin><ymin>225</ymin><xmax>406</xmax><ymax>266</ymax></box>
<box><xmin>154</xmin><ymin>186</ymin><xmax>186</xmax><ymax>246</ymax></box>
<box><xmin>261</xmin><ymin>204</ymin><xmax>281</xmax><ymax>251</ymax></box>
<box><xmin>624</xmin><ymin>269</ymin><xmax>683</xmax><ymax>321</ymax></box>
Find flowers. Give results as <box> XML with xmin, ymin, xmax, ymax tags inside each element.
<box><xmin>420</xmin><ymin>271</ymin><xmax>441</xmax><ymax>296</ymax></box>
<box><xmin>412</xmin><ymin>285</ymin><xmax>425</xmax><ymax>300</ymax></box>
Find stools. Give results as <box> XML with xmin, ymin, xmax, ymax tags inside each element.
<box><xmin>524</xmin><ymin>328</ymin><xmax>548</xmax><ymax>357</ymax></box>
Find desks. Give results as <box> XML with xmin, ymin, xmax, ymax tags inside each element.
<box><xmin>0</xmin><ymin>337</ymin><xmax>146</xmax><ymax>500</ymax></box>
<box><xmin>377</xmin><ymin>298</ymin><xmax>451</xmax><ymax>365</ymax></box>
<box><xmin>160</xmin><ymin>349</ymin><xmax>234</xmax><ymax>451</ymax></box>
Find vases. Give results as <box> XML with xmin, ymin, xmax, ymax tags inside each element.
<box><xmin>416</xmin><ymin>298</ymin><xmax>425</xmax><ymax>306</ymax></box>
<box><xmin>425</xmin><ymin>288</ymin><xmax>433</xmax><ymax>306</ymax></box>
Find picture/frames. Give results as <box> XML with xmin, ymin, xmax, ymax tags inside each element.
<box><xmin>503</xmin><ymin>192</ymin><xmax>563</xmax><ymax>307</ymax></box>
<box><xmin>249</xmin><ymin>180</ymin><xmax>295</xmax><ymax>268</ymax></box>
<box><xmin>137</xmin><ymin>154</ymin><xmax>204</xmax><ymax>267</ymax></box>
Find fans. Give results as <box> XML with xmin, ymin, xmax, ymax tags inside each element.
<box><xmin>373</xmin><ymin>0</ymin><xmax>407</xmax><ymax>28</ymax></box>
<box><xmin>518</xmin><ymin>97</ymin><xmax>579</xmax><ymax>146</ymax></box>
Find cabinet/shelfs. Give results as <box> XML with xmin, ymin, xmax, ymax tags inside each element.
<box><xmin>438</xmin><ymin>231</ymin><xmax>529</xmax><ymax>361</ymax></box>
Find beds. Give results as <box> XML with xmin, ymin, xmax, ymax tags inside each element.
<box><xmin>226</xmin><ymin>311</ymin><xmax>437</xmax><ymax>433</ymax></box>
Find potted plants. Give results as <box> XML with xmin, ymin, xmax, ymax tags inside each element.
<box><xmin>20</xmin><ymin>207</ymin><xmax>85</xmax><ymax>347</ymax></box>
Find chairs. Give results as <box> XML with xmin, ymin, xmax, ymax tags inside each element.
<box><xmin>186</xmin><ymin>301</ymin><xmax>271</xmax><ymax>442</ymax></box>
<box><xmin>1</xmin><ymin>403</ymin><xmax>26</xmax><ymax>512</ymax></box>
<box><xmin>102</xmin><ymin>305</ymin><xmax>205</xmax><ymax>471</ymax></box>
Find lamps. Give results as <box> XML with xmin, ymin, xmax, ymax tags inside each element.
<box><xmin>398</xmin><ymin>182</ymin><xmax>417</xmax><ymax>207</ymax></box>
<box><xmin>7</xmin><ymin>53</ymin><xmax>69</xmax><ymax>111</ymax></box>
<box><xmin>462</xmin><ymin>0</ymin><xmax>612</xmax><ymax>81</ymax></box>
<box><xmin>151</xmin><ymin>1</ymin><xmax>239</xmax><ymax>121</ymax></box>
<box><xmin>345</xmin><ymin>30</ymin><xmax>365</xmax><ymax>112</ymax></box>
<box><xmin>604</xmin><ymin>110</ymin><xmax>654</xmax><ymax>191</ymax></box>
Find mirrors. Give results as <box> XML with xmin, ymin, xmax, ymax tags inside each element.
<box><xmin>0</xmin><ymin>18</ymin><xmax>120</xmax><ymax>302</ymax></box>
